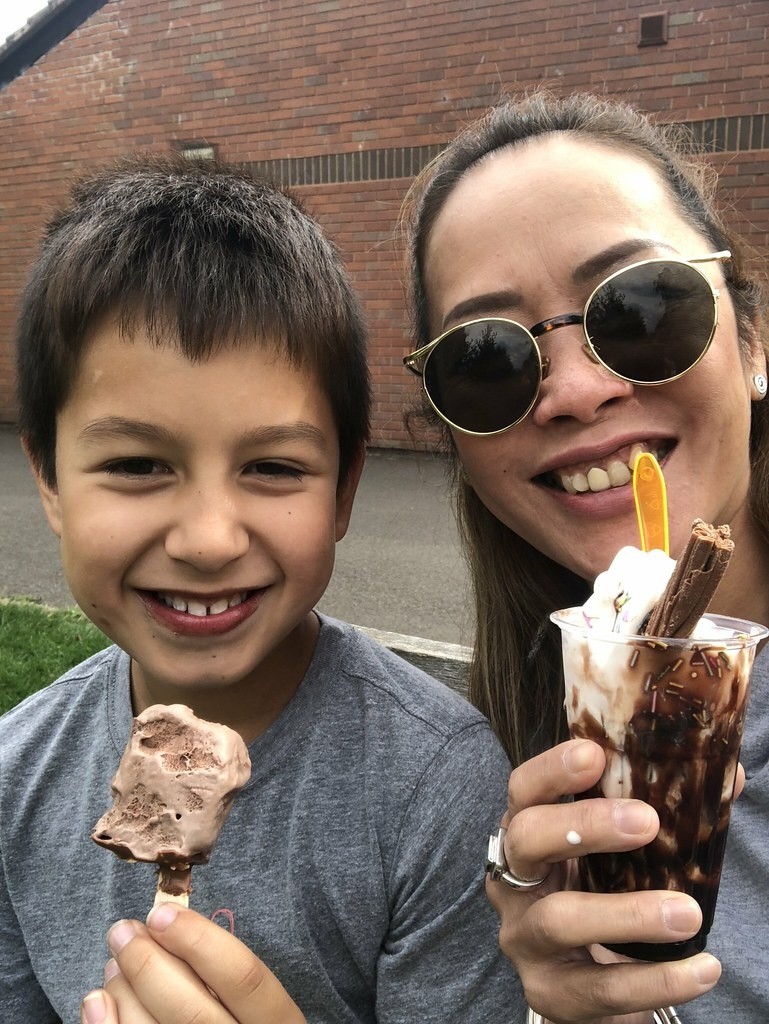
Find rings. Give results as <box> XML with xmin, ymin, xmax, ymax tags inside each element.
<box><xmin>485</xmin><ymin>827</ymin><xmax>551</xmax><ymax>892</ymax></box>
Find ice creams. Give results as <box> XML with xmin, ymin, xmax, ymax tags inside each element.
<box><xmin>552</xmin><ymin>522</ymin><xmax>769</xmax><ymax>964</ymax></box>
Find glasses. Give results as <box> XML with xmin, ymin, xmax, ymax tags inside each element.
<box><xmin>402</xmin><ymin>251</ymin><xmax>733</xmax><ymax>437</ymax></box>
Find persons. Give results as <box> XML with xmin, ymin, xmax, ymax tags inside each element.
<box><xmin>0</xmin><ymin>157</ymin><xmax>527</xmax><ymax>1024</ymax></box>
<box><xmin>399</xmin><ymin>88</ymin><xmax>769</xmax><ymax>1024</ymax></box>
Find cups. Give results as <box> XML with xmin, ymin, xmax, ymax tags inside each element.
<box><xmin>550</xmin><ymin>605</ymin><xmax>769</xmax><ymax>962</ymax></box>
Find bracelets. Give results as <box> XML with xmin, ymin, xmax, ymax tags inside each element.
<box><xmin>527</xmin><ymin>1006</ymin><xmax>681</xmax><ymax>1024</ymax></box>
